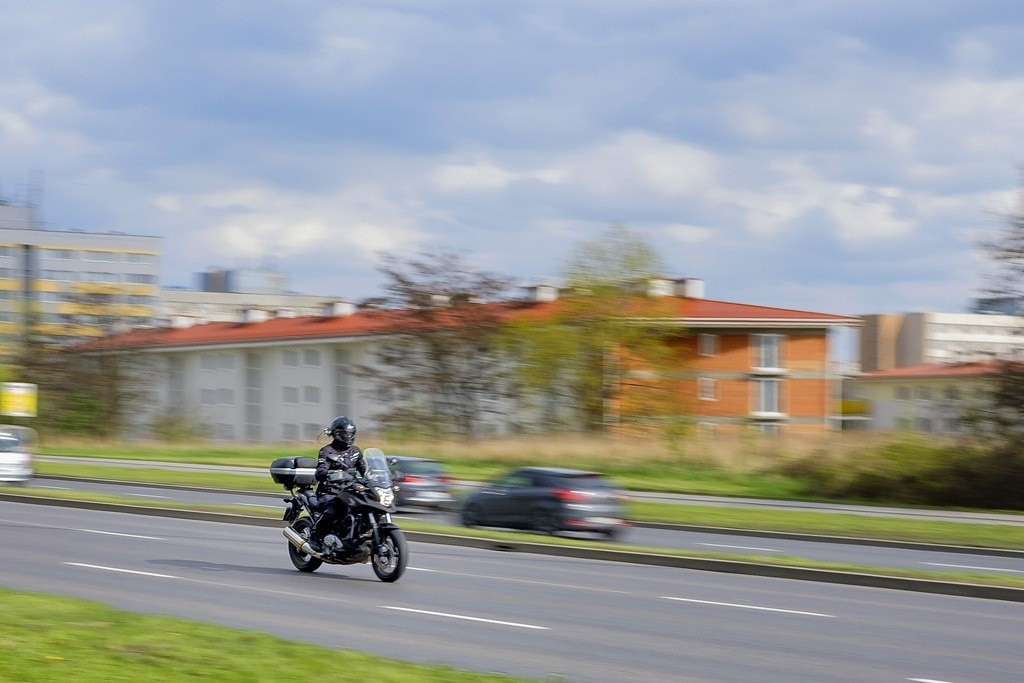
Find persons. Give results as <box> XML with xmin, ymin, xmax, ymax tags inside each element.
<box><xmin>308</xmin><ymin>415</ymin><xmax>370</xmax><ymax>552</ymax></box>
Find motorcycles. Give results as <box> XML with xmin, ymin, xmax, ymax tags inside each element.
<box><xmin>267</xmin><ymin>446</ymin><xmax>412</xmax><ymax>584</ymax></box>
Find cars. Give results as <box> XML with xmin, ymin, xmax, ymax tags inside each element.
<box><xmin>0</xmin><ymin>430</ymin><xmax>36</xmax><ymax>484</ymax></box>
<box><xmin>462</xmin><ymin>466</ymin><xmax>639</xmax><ymax>540</ymax></box>
<box><xmin>383</xmin><ymin>457</ymin><xmax>455</xmax><ymax>515</ymax></box>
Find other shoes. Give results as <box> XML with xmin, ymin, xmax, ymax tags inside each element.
<box><xmin>308</xmin><ymin>533</ymin><xmax>322</xmax><ymax>554</ymax></box>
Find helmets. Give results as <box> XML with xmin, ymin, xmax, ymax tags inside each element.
<box><xmin>329</xmin><ymin>417</ymin><xmax>356</xmax><ymax>449</ymax></box>
